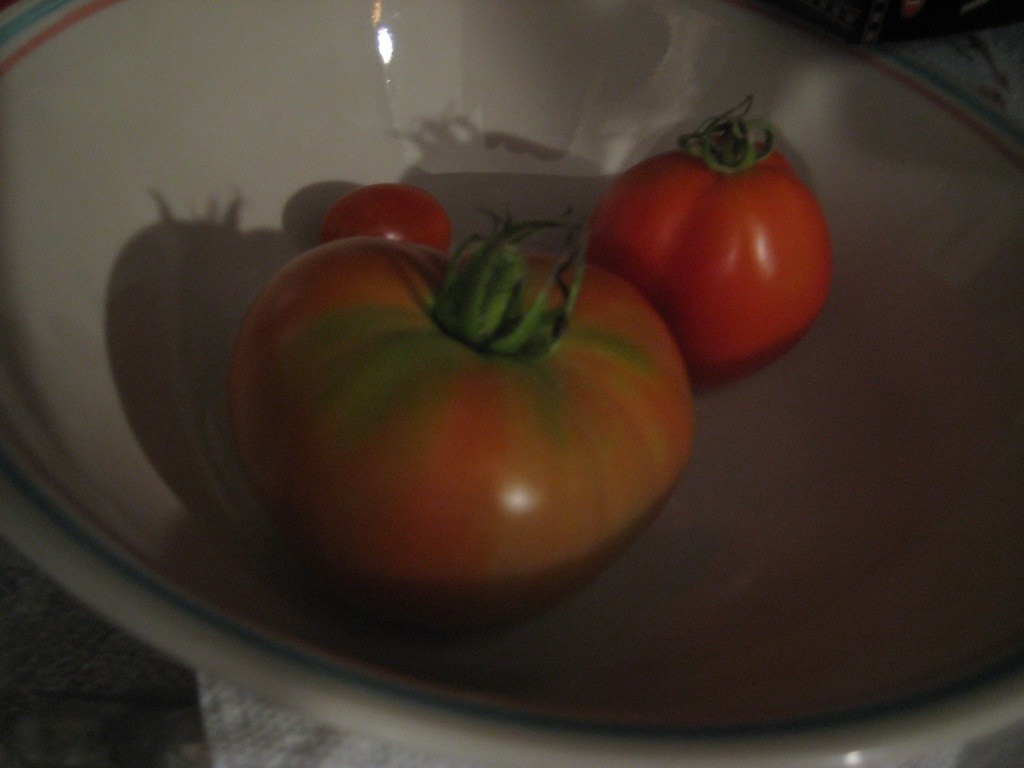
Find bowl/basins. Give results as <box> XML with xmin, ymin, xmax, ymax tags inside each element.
<box><xmin>1</xmin><ymin>0</ymin><xmax>1023</xmax><ymax>768</ymax></box>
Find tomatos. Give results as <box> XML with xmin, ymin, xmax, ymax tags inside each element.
<box><xmin>228</xmin><ymin>132</ymin><xmax>832</xmax><ymax>626</ymax></box>
